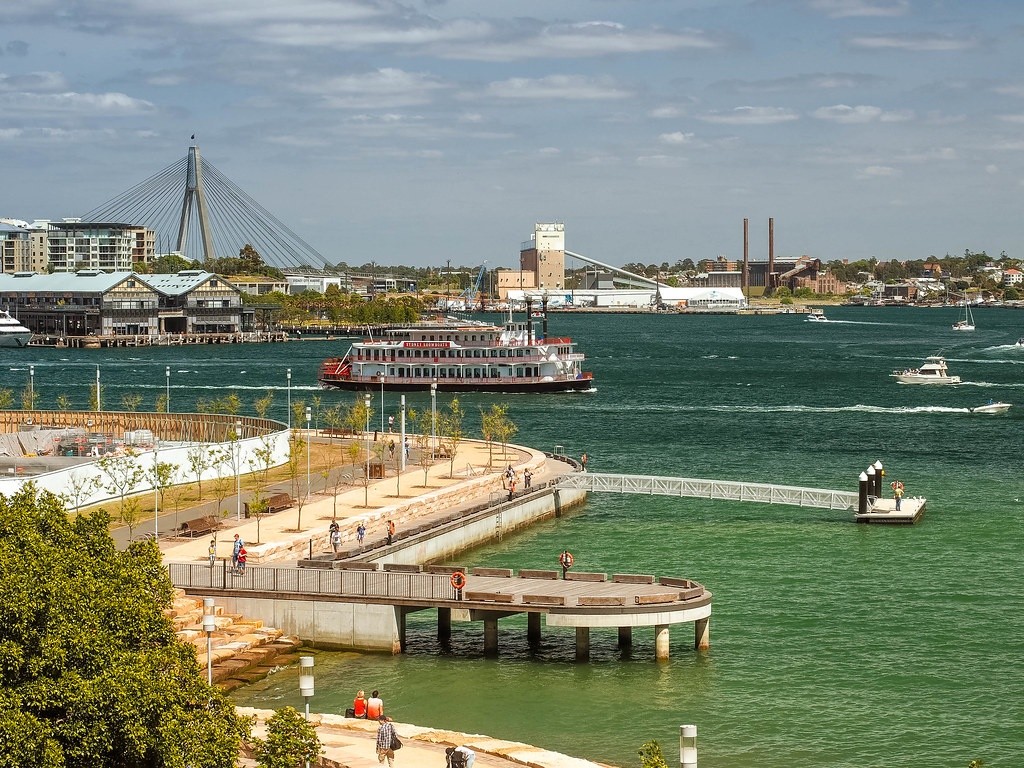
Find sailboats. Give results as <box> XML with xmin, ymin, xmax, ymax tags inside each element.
<box><xmin>951</xmin><ymin>289</ymin><xmax>978</xmax><ymax>332</ymax></box>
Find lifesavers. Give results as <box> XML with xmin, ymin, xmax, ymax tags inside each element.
<box><xmin>377</xmin><ymin>371</ymin><xmax>381</xmax><ymax>376</ymax></box>
<box><xmin>451</xmin><ymin>572</ymin><xmax>465</xmax><ymax>589</ymax></box>
<box><xmin>559</xmin><ymin>553</ymin><xmax>573</xmax><ymax>569</ymax></box>
<box><xmin>465</xmin><ymin>298</ymin><xmax>469</xmax><ymax>303</ymax></box>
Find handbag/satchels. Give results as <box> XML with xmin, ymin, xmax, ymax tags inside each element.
<box><xmin>390</xmin><ymin>733</ymin><xmax>403</xmax><ymax>750</ymax></box>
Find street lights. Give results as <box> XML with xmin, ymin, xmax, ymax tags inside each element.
<box><xmin>305</xmin><ymin>406</ymin><xmax>314</xmax><ymax>499</ymax></box>
<box><xmin>95</xmin><ymin>363</ymin><xmax>101</xmax><ymax>411</ymax></box>
<box><xmin>371</xmin><ymin>261</ymin><xmax>375</xmax><ymax>302</ymax></box>
<box><xmin>430</xmin><ymin>376</ymin><xmax>437</xmax><ymax>462</ymax></box>
<box><xmin>344</xmin><ymin>269</ymin><xmax>348</xmax><ymax>299</ymax></box>
<box><xmin>381</xmin><ymin>371</ymin><xmax>386</xmax><ymax>442</ymax></box>
<box><xmin>399</xmin><ymin>393</ymin><xmax>407</xmax><ymax>471</ymax></box>
<box><xmin>364</xmin><ymin>393</ymin><xmax>369</xmax><ymax>507</ymax></box>
<box><xmin>416</xmin><ymin>269</ymin><xmax>420</xmax><ymax>299</ymax></box>
<box><xmin>285</xmin><ymin>368</ymin><xmax>291</xmax><ymax>428</ymax></box>
<box><xmin>298</xmin><ymin>656</ymin><xmax>316</xmax><ymax>767</ymax></box>
<box><xmin>202</xmin><ymin>598</ymin><xmax>214</xmax><ymax>690</ymax></box>
<box><xmin>30</xmin><ymin>366</ymin><xmax>35</xmax><ymax>410</ymax></box>
<box><xmin>165</xmin><ymin>366</ymin><xmax>171</xmax><ymax>413</ymax></box>
<box><xmin>446</xmin><ymin>259</ymin><xmax>452</xmax><ymax>299</ymax></box>
<box><xmin>235</xmin><ymin>421</ymin><xmax>242</xmax><ymax>521</ymax></box>
<box><xmin>152</xmin><ymin>436</ymin><xmax>160</xmax><ymax>544</ymax></box>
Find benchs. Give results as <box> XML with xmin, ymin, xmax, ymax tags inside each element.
<box><xmin>260</xmin><ymin>493</ymin><xmax>297</xmax><ymax>514</ymax></box>
<box><xmin>319</xmin><ymin>428</ymin><xmax>361</xmax><ymax>439</ymax></box>
<box><xmin>170</xmin><ymin>515</ymin><xmax>223</xmax><ymax>537</ymax></box>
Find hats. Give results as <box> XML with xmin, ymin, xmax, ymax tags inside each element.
<box><xmin>377</xmin><ymin>715</ymin><xmax>386</xmax><ymax>720</ymax></box>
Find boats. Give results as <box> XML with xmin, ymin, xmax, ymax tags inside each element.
<box><xmin>317</xmin><ymin>290</ymin><xmax>594</xmax><ymax>393</ymax></box>
<box><xmin>806</xmin><ymin>313</ymin><xmax>828</xmax><ymax>323</ymax></box>
<box><xmin>970</xmin><ymin>397</ymin><xmax>1023</xmax><ymax>418</ymax></box>
<box><xmin>890</xmin><ymin>346</ymin><xmax>966</xmax><ymax>388</ymax></box>
<box><xmin>531</xmin><ymin>311</ymin><xmax>545</xmax><ymax>318</ymax></box>
<box><xmin>1016</xmin><ymin>338</ymin><xmax>1023</xmax><ymax>348</ymax></box>
<box><xmin>0</xmin><ymin>310</ymin><xmax>32</xmax><ymax>349</ymax></box>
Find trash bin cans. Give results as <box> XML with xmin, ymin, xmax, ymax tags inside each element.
<box><xmin>365</xmin><ymin>462</ymin><xmax>374</xmax><ymax>479</ymax></box>
<box><xmin>374</xmin><ymin>462</ymin><xmax>385</xmax><ymax>479</ymax></box>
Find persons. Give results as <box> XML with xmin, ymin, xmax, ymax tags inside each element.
<box><xmin>232</xmin><ymin>534</ymin><xmax>244</xmax><ymax>573</ymax></box>
<box><xmin>330</xmin><ymin>517</ymin><xmax>343</xmax><ymax>552</ymax></box>
<box><xmin>902</xmin><ymin>366</ymin><xmax>920</xmax><ymax>376</ymax></box>
<box><xmin>388</xmin><ymin>440</ymin><xmax>395</xmax><ymax>460</ymax></box>
<box><xmin>446</xmin><ymin>746</ymin><xmax>476</xmax><ymax>768</ymax></box>
<box><xmin>405</xmin><ymin>437</ymin><xmax>410</xmax><ymax>459</ymax></box>
<box><xmin>524</xmin><ymin>468</ymin><xmax>532</xmax><ymax>488</ymax></box>
<box><xmin>375</xmin><ymin>715</ymin><xmax>398</xmax><ymax>768</ymax></box>
<box><xmin>352</xmin><ymin>689</ymin><xmax>367</xmax><ymax>719</ymax></box>
<box><xmin>236</xmin><ymin>544</ymin><xmax>247</xmax><ymax>575</ymax></box>
<box><xmin>388</xmin><ymin>413</ymin><xmax>395</xmax><ymax>434</ymax></box>
<box><xmin>208</xmin><ymin>540</ymin><xmax>216</xmax><ymax>566</ymax></box>
<box><xmin>357</xmin><ymin>523</ymin><xmax>366</xmax><ymax>548</ymax></box>
<box><xmin>367</xmin><ymin>690</ymin><xmax>393</xmax><ymax>721</ymax></box>
<box><xmin>582</xmin><ymin>453</ymin><xmax>586</xmax><ymax>470</ymax></box>
<box><xmin>505</xmin><ymin>465</ymin><xmax>514</xmax><ymax>483</ymax></box>
<box><xmin>894</xmin><ymin>486</ymin><xmax>904</xmax><ymax>512</ymax></box>
